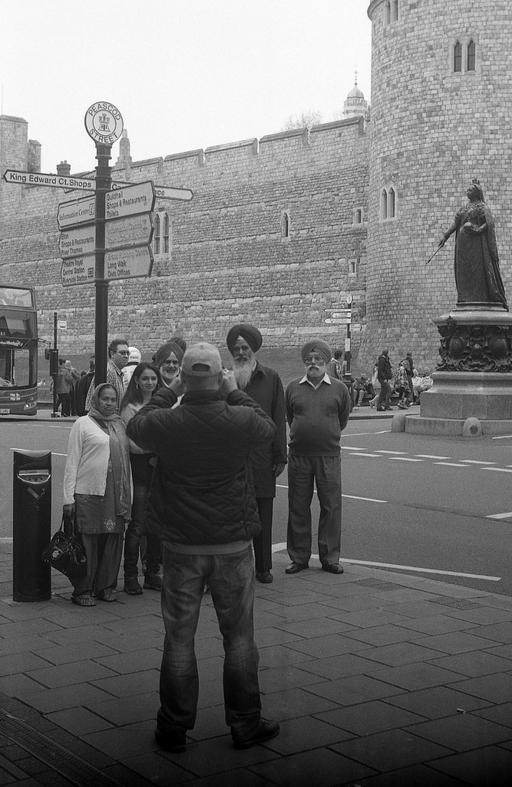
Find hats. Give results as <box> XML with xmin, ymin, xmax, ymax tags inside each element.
<box><xmin>301</xmin><ymin>339</ymin><xmax>332</xmax><ymax>365</ymax></box>
<box><xmin>126</xmin><ymin>348</ymin><xmax>141</xmax><ymax>363</ymax></box>
<box><xmin>151</xmin><ymin>342</ymin><xmax>183</xmax><ymax>368</ymax></box>
<box><xmin>226</xmin><ymin>323</ymin><xmax>262</xmax><ymax>353</ymax></box>
<box><xmin>181</xmin><ymin>342</ymin><xmax>222</xmax><ymax>377</ymax></box>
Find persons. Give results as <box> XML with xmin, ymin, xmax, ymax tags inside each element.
<box><xmin>126</xmin><ymin>342</ymin><xmax>280</xmax><ymax>751</ymax></box>
<box><xmin>440</xmin><ymin>180</ymin><xmax>510</xmax><ymax>314</ymax></box>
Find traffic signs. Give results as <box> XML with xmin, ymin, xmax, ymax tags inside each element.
<box><xmin>332</xmin><ymin>312</ymin><xmax>352</xmax><ymax>318</ymax></box>
<box><xmin>57</xmin><ymin>212</ymin><xmax>154</xmax><ymax>260</ymax></box>
<box><xmin>109</xmin><ymin>180</ymin><xmax>195</xmax><ymax>202</ymax></box>
<box><xmin>2</xmin><ymin>169</ymin><xmax>97</xmax><ymax>191</ymax></box>
<box><xmin>325</xmin><ymin>308</ymin><xmax>351</xmax><ymax>312</ymax></box>
<box><xmin>325</xmin><ymin>318</ymin><xmax>351</xmax><ymax>323</ymax></box>
<box><xmin>59</xmin><ymin>244</ymin><xmax>156</xmax><ymax>288</ymax></box>
<box><xmin>55</xmin><ymin>180</ymin><xmax>155</xmax><ymax>230</ymax></box>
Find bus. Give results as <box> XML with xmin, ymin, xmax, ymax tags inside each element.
<box><xmin>0</xmin><ymin>281</ymin><xmax>51</xmax><ymax>417</ymax></box>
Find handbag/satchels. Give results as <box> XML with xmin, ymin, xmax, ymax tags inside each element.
<box><xmin>50</xmin><ymin>512</ymin><xmax>87</xmax><ymax>578</ymax></box>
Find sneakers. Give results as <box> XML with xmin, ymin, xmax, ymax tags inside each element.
<box><xmin>97</xmin><ymin>594</ymin><xmax>117</xmax><ymax>602</ymax></box>
<box><xmin>143</xmin><ymin>577</ymin><xmax>162</xmax><ymax>591</ymax></box>
<box><xmin>321</xmin><ymin>564</ymin><xmax>344</xmax><ymax>575</ymax></box>
<box><xmin>255</xmin><ymin>571</ymin><xmax>273</xmax><ymax>583</ymax></box>
<box><xmin>228</xmin><ymin>717</ymin><xmax>279</xmax><ymax>749</ymax></box>
<box><xmin>71</xmin><ymin>595</ymin><xmax>96</xmax><ymax>607</ymax></box>
<box><xmin>156</xmin><ymin>735</ymin><xmax>188</xmax><ymax>754</ymax></box>
<box><xmin>123</xmin><ymin>579</ymin><xmax>142</xmax><ymax>595</ymax></box>
<box><xmin>285</xmin><ymin>563</ymin><xmax>309</xmax><ymax>574</ymax></box>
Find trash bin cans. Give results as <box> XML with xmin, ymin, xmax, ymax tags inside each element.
<box><xmin>13</xmin><ymin>450</ymin><xmax>52</xmax><ymax>602</ymax></box>
<box><xmin>342</xmin><ymin>373</ymin><xmax>354</xmax><ymax>412</ymax></box>
